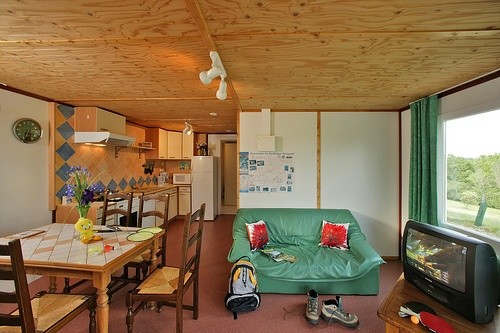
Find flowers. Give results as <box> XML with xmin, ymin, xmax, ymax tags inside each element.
<box><xmin>57</xmin><ymin>168</ymin><xmax>111</xmax><ymax>206</ymax></box>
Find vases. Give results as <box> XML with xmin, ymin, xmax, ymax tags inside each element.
<box><xmin>76</xmin><ymin>206</ymin><xmax>91</xmax><ymax>218</ymax></box>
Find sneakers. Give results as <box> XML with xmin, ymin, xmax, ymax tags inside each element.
<box><xmin>321</xmin><ymin>295</ymin><xmax>360</xmax><ymax>327</ymax></box>
<box><xmin>305</xmin><ymin>288</ymin><xmax>320</xmax><ymax>326</ymax></box>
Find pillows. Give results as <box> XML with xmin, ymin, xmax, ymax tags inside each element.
<box><xmin>320</xmin><ymin>219</ymin><xmax>350</xmax><ymax>251</ymax></box>
<box><xmin>245</xmin><ymin>219</ymin><xmax>269</xmax><ymax>252</ymax></box>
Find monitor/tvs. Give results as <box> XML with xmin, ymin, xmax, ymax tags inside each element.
<box><xmin>402</xmin><ymin>220</ymin><xmax>500</xmax><ymax>324</ymax></box>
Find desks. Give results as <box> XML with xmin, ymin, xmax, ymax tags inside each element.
<box><xmin>0</xmin><ymin>222</ymin><xmax>166</xmax><ymax>333</ymax></box>
<box><xmin>376</xmin><ymin>271</ymin><xmax>500</xmax><ymax>333</ymax></box>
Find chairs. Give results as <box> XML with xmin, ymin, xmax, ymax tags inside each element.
<box><xmin>101</xmin><ymin>189</ymin><xmax>206</xmax><ymax>333</ymax></box>
<box><xmin>0</xmin><ymin>238</ymin><xmax>98</xmax><ymax>333</ymax></box>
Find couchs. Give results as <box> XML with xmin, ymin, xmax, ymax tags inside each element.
<box><xmin>227</xmin><ymin>208</ymin><xmax>387</xmax><ymax>294</ymax></box>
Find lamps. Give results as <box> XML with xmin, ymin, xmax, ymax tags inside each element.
<box><xmin>199</xmin><ymin>60</ymin><xmax>227</xmax><ymax>101</ymax></box>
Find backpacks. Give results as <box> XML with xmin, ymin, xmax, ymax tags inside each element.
<box><xmin>224</xmin><ymin>256</ymin><xmax>261</xmax><ymax>320</ymax></box>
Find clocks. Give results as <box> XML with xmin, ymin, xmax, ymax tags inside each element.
<box><xmin>12</xmin><ymin>118</ymin><xmax>43</xmax><ymax>145</ymax></box>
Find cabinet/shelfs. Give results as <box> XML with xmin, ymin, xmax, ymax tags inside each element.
<box><xmin>55</xmin><ymin>184</ymin><xmax>192</xmax><ymax>230</ymax></box>
<box><xmin>75</xmin><ymin>107</ymin><xmax>137</xmax><ymax>136</ymax></box>
<box><xmin>183</xmin><ymin>132</ymin><xmax>194</xmax><ymax>160</ymax></box>
<box><xmin>145</xmin><ymin>127</ymin><xmax>182</xmax><ymax>160</ymax></box>
